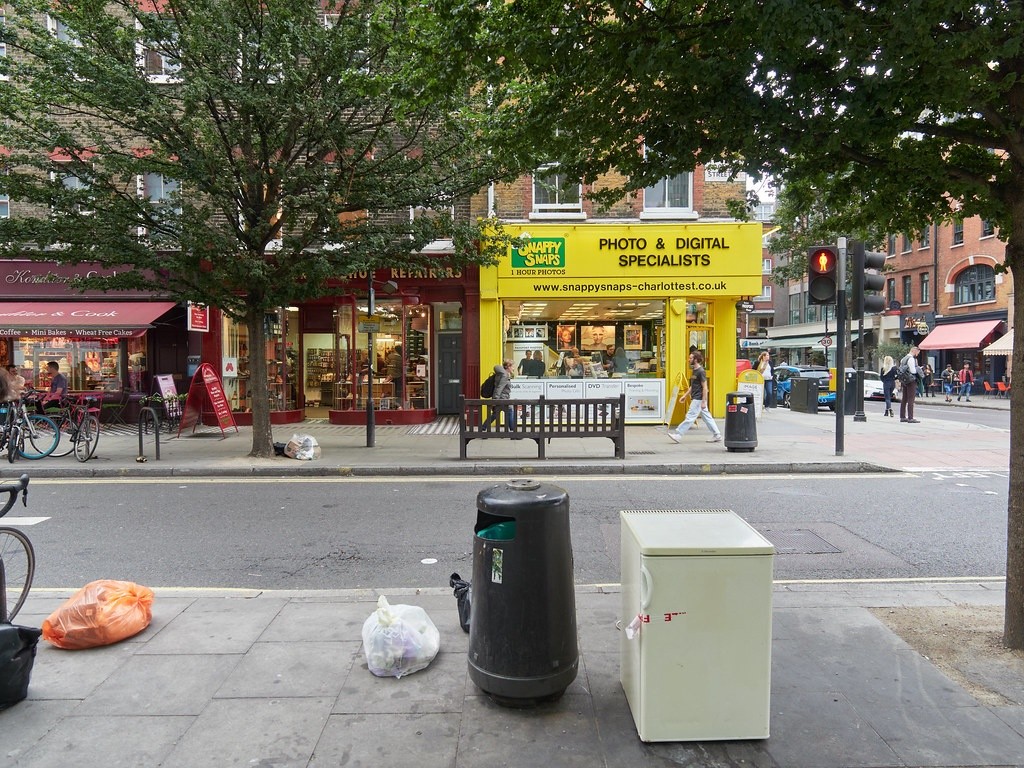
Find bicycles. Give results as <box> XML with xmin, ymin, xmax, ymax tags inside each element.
<box><xmin>0</xmin><ymin>473</ymin><xmax>36</xmax><ymax>626</ymax></box>
<box><xmin>0</xmin><ymin>388</ymin><xmax>100</xmax><ymax>462</ymax></box>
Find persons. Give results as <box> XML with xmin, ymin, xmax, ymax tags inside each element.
<box><xmin>919</xmin><ymin>362</ymin><xmax>975</xmax><ymax>403</ymax></box>
<box><xmin>558</xmin><ymin>329</ymin><xmax>575</xmax><ymax>349</ymax></box>
<box><xmin>377</xmin><ymin>345</ymin><xmax>405</xmax><ymax>403</ymax></box>
<box><xmin>584</xmin><ymin>325</ymin><xmax>611</xmax><ymax>350</ymax></box>
<box><xmin>0</xmin><ymin>364</ymin><xmax>27</xmax><ymax>409</ymax></box>
<box><xmin>36</xmin><ymin>360</ymin><xmax>68</xmax><ymax>428</ymax></box>
<box><xmin>880</xmin><ymin>355</ymin><xmax>899</xmax><ymax>417</ymax></box>
<box><xmin>751</xmin><ymin>352</ymin><xmax>776</xmax><ymax>412</ymax></box>
<box><xmin>480</xmin><ymin>359</ymin><xmax>524</xmax><ymax>440</ymax></box>
<box><xmin>563</xmin><ymin>343</ymin><xmax>630</xmax><ymax>377</ymax></box>
<box><xmin>516</xmin><ymin>349</ymin><xmax>547</xmax><ymax>378</ymax></box>
<box><xmin>780</xmin><ymin>359</ymin><xmax>788</xmax><ymax>366</ymax></box>
<box><xmin>897</xmin><ymin>346</ymin><xmax>925</xmax><ymax>422</ymax></box>
<box><xmin>668</xmin><ymin>350</ymin><xmax>722</xmax><ymax>443</ymax></box>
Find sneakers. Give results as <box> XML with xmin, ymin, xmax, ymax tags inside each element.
<box><xmin>762</xmin><ymin>406</ymin><xmax>766</xmax><ymax>414</ymax></box>
<box><xmin>668</xmin><ymin>433</ymin><xmax>682</xmax><ymax>443</ymax></box>
<box><xmin>705</xmin><ymin>434</ymin><xmax>723</xmax><ymax>443</ymax></box>
<box><xmin>765</xmin><ymin>407</ymin><xmax>771</xmax><ymax>413</ymax></box>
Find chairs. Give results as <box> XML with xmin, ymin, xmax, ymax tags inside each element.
<box><xmin>983</xmin><ymin>382</ymin><xmax>997</xmax><ymax>399</ymax></box>
<box><xmin>997</xmin><ymin>382</ymin><xmax>1008</xmax><ymax>399</ymax></box>
<box><xmin>26</xmin><ymin>387</ymin><xmax>132</xmax><ymax>430</ymax></box>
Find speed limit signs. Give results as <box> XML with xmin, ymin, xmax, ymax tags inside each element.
<box><xmin>821</xmin><ymin>337</ymin><xmax>833</xmax><ymax>346</ymax></box>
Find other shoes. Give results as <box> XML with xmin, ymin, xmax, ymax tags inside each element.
<box><xmin>966</xmin><ymin>399</ymin><xmax>971</xmax><ymax>402</ymax></box>
<box><xmin>932</xmin><ymin>394</ymin><xmax>936</xmax><ymax>397</ymax></box>
<box><xmin>957</xmin><ymin>396</ymin><xmax>961</xmax><ymax>401</ymax></box>
<box><xmin>926</xmin><ymin>394</ymin><xmax>929</xmax><ymax>397</ymax></box>
<box><xmin>945</xmin><ymin>399</ymin><xmax>952</xmax><ymax>402</ymax></box>
<box><xmin>900</xmin><ymin>417</ymin><xmax>908</xmax><ymax>422</ymax></box>
<box><xmin>884</xmin><ymin>410</ymin><xmax>888</xmax><ymax>416</ymax></box>
<box><xmin>908</xmin><ymin>418</ymin><xmax>920</xmax><ymax>423</ymax></box>
<box><xmin>890</xmin><ymin>409</ymin><xmax>894</xmax><ymax>417</ymax></box>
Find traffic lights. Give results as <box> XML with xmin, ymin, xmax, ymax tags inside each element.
<box><xmin>808</xmin><ymin>246</ymin><xmax>837</xmax><ymax>305</ymax></box>
<box><xmin>848</xmin><ymin>240</ymin><xmax>887</xmax><ymax>316</ymax></box>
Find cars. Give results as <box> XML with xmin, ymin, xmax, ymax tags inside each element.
<box><xmin>863</xmin><ymin>370</ymin><xmax>899</xmax><ymax>402</ymax></box>
<box><xmin>764</xmin><ymin>364</ymin><xmax>837</xmax><ymax>411</ymax></box>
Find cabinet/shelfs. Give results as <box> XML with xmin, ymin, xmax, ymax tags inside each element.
<box><xmin>408</xmin><ymin>382</ymin><xmax>426</xmax><ymax>398</ymax></box>
<box><xmin>335</xmin><ymin>383</ymin><xmax>398</xmax><ymax>411</ymax></box>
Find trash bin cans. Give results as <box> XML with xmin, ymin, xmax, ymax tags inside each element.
<box><xmin>724</xmin><ymin>392</ymin><xmax>759</xmax><ymax>453</ymax></box>
<box><xmin>466</xmin><ymin>479</ymin><xmax>582</xmax><ymax>709</ymax></box>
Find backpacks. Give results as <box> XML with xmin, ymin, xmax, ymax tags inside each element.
<box><xmin>897</xmin><ymin>356</ymin><xmax>916</xmax><ymax>384</ymax></box>
<box><xmin>481</xmin><ymin>372</ymin><xmax>504</xmax><ymax>398</ymax></box>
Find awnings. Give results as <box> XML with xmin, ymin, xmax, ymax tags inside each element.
<box><xmin>917</xmin><ymin>319</ymin><xmax>1015</xmax><ymax>356</ymax></box>
<box><xmin>0</xmin><ymin>301</ymin><xmax>177</xmax><ymax>338</ymax></box>
<box><xmin>760</xmin><ymin>332</ymin><xmax>870</xmax><ymax>350</ymax></box>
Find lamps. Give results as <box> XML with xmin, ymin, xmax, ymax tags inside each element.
<box><xmin>913</xmin><ymin>330</ymin><xmax>918</xmax><ymax>335</ymax></box>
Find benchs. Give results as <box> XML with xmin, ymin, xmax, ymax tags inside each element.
<box><xmin>459</xmin><ymin>394</ymin><xmax>625</xmax><ymax>460</ymax></box>
